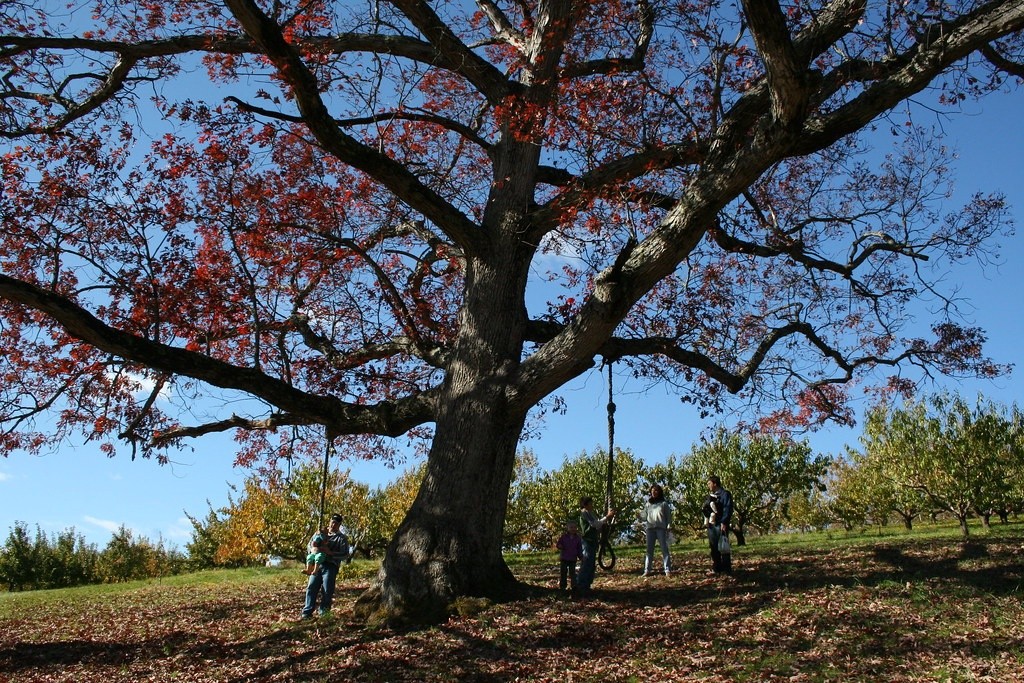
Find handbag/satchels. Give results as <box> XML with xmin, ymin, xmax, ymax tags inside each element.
<box><xmin>718</xmin><ymin>530</ymin><xmax>730</xmax><ymax>554</ymax></box>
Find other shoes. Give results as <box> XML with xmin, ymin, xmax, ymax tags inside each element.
<box><xmin>666</xmin><ymin>572</ymin><xmax>672</xmax><ymax>580</ymax></box>
<box><xmin>311</xmin><ymin>571</ymin><xmax>319</xmax><ymax>577</ymax></box>
<box><xmin>301</xmin><ymin>570</ymin><xmax>311</xmax><ymax>575</ymax></box>
<box><xmin>301</xmin><ymin>613</ymin><xmax>312</xmax><ymax>620</ymax></box>
<box><xmin>638</xmin><ymin>572</ymin><xmax>650</xmax><ymax>579</ymax></box>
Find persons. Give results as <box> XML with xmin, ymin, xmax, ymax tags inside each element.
<box><xmin>633</xmin><ymin>484</ymin><xmax>673</xmax><ymax>578</ymax></box>
<box><xmin>301</xmin><ymin>527</ymin><xmax>328</xmax><ymax>576</ymax></box>
<box><xmin>558</xmin><ymin>520</ymin><xmax>585</xmax><ymax>589</ymax></box>
<box><xmin>571</xmin><ymin>496</ymin><xmax>616</xmax><ymax>600</ymax></box>
<box><xmin>300</xmin><ymin>513</ymin><xmax>349</xmax><ymax>620</ymax></box>
<box><xmin>702</xmin><ymin>493</ymin><xmax>718</xmax><ymax>526</ymax></box>
<box><xmin>701</xmin><ymin>476</ymin><xmax>734</xmax><ymax>577</ymax></box>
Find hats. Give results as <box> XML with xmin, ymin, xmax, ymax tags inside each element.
<box><xmin>331</xmin><ymin>514</ymin><xmax>342</xmax><ymax>523</ymax></box>
